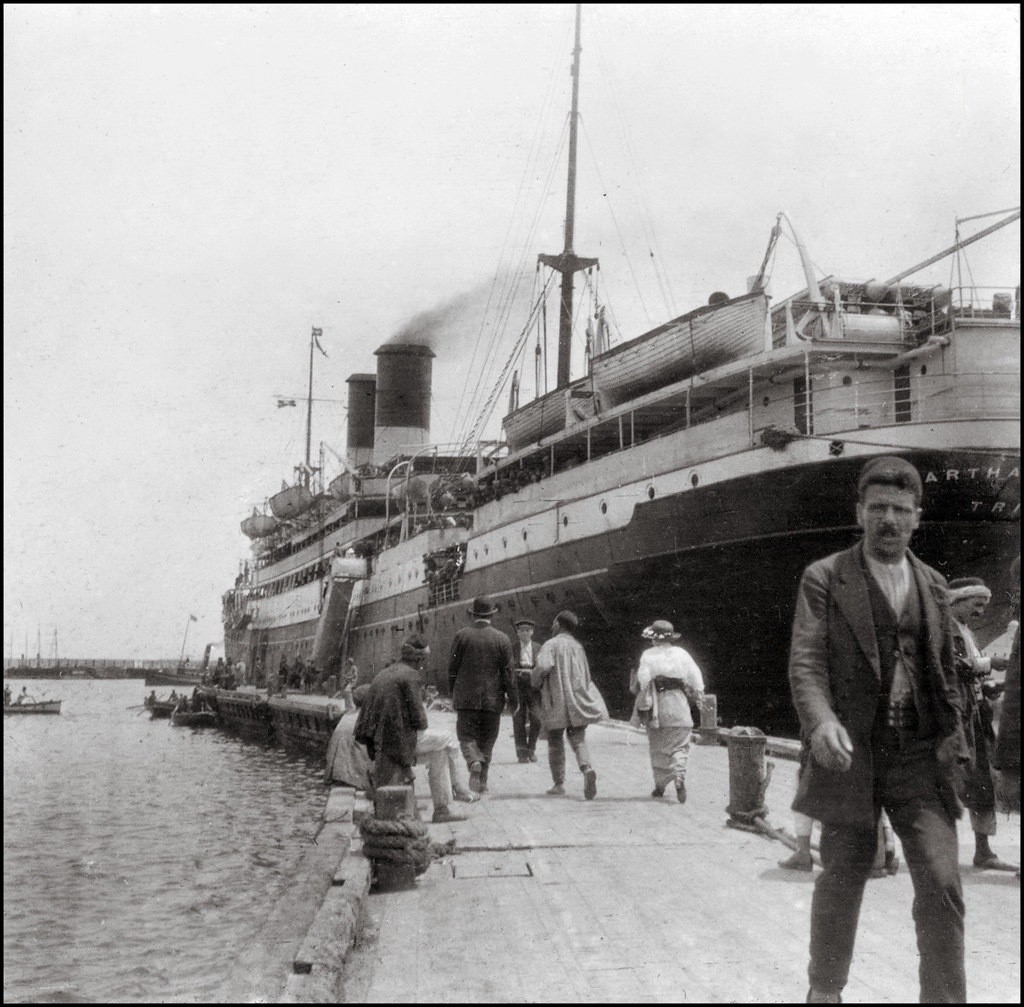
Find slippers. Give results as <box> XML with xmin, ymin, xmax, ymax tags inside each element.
<box><xmin>972</xmin><ymin>852</ymin><xmax>1020</xmax><ymax>875</ymax></box>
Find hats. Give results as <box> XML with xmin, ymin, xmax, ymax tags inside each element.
<box><xmin>468</xmin><ymin>595</ymin><xmax>498</xmax><ymax>615</ymax></box>
<box><xmin>400</xmin><ymin>637</ymin><xmax>431</xmax><ymax>661</ymax></box>
<box><xmin>944</xmin><ymin>575</ymin><xmax>991</xmax><ymax>606</ymax></box>
<box><xmin>640</xmin><ymin>620</ymin><xmax>682</xmax><ymax>641</ymax></box>
<box><xmin>514</xmin><ymin>615</ymin><xmax>535</xmax><ymax>628</ymax></box>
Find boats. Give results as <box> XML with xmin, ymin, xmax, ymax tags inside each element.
<box><xmin>588</xmin><ymin>288</ymin><xmax>772</xmax><ymax>411</ymax></box>
<box><xmin>268</xmin><ymin>483</ymin><xmax>313</xmax><ymax>521</ymax></box>
<box><xmin>327</xmin><ymin>462</ymin><xmax>377</xmax><ymax>502</ymax></box>
<box><xmin>500</xmin><ymin>374</ymin><xmax>598</xmax><ymax>452</ymax></box>
<box><xmin>240</xmin><ymin>514</ymin><xmax>278</xmax><ymax>540</ymax></box>
<box><xmin>170</xmin><ymin>698</ymin><xmax>217</xmax><ymax>728</ymax></box>
<box><xmin>4</xmin><ymin>699</ymin><xmax>63</xmax><ymax>715</ymax></box>
<box><xmin>142</xmin><ymin>700</ymin><xmax>178</xmax><ymax>720</ymax></box>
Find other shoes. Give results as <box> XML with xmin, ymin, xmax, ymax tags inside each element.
<box><xmin>431</xmin><ymin>808</ymin><xmax>468</xmax><ymax>823</ymax></box>
<box><xmin>867</xmin><ymin>857</ymin><xmax>888</xmax><ymax>877</ymax></box>
<box><xmin>547</xmin><ymin>786</ymin><xmax>567</xmax><ymax>795</ymax></box>
<box><xmin>779</xmin><ymin>853</ymin><xmax>811</xmax><ymax>872</ymax></box>
<box><xmin>469</xmin><ymin>761</ymin><xmax>488</xmax><ymax>794</ymax></box>
<box><xmin>455</xmin><ymin>790</ymin><xmax>481</xmax><ymax>803</ymax></box>
<box><xmin>583</xmin><ymin>767</ymin><xmax>597</xmax><ymax>801</ymax></box>
<box><xmin>652</xmin><ymin>787</ymin><xmax>664</xmax><ymax>797</ymax></box>
<box><xmin>518</xmin><ymin>746</ymin><xmax>537</xmax><ymax>763</ymax></box>
<box><xmin>673</xmin><ymin>775</ymin><xmax>687</xmax><ymax>803</ymax></box>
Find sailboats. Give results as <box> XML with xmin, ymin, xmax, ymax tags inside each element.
<box><xmin>143</xmin><ymin>615</ymin><xmax>203</xmax><ymax>687</ymax></box>
<box><xmin>220</xmin><ymin>7</ymin><xmax>1018</xmax><ymax>720</ymax></box>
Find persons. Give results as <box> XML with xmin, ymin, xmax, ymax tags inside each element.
<box><xmin>353</xmin><ymin>634</ymin><xmax>480</xmax><ymax>823</ymax></box>
<box><xmin>147</xmin><ymin>682</ymin><xmax>221</xmax><ymax>716</ymax></box>
<box><xmin>531</xmin><ymin>611</ymin><xmax>611</xmax><ymax>800</ymax></box>
<box><xmin>3</xmin><ymin>682</ymin><xmax>34</xmax><ymax>706</ymax></box>
<box><xmin>777</xmin><ymin>456</ymin><xmax>970</xmax><ymax>1007</ymax></box>
<box><xmin>950</xmin><ymin>580</ymin><xmax>1022</xmax><ymax>871</ymax></box>
<box><xmin>215</xmin><ymin>656</ymin><xmax>246</xmax><ymax>685</ymax></box>
<box><xmin>628</xmin><ymin>620</ymin><xmax>704</xmax><ymax>804</ymax></box>
<box><xmin>278</xmin><ymin>654</ymin><xmax>330</xmax><ymax>692</ymax></box>
<box><xmin>509</xmin><ymin>620</ymin><xmax>545</xmax><ymax>762</ymax></box>
<box><xmin>448</xmin><ymin>594</ymin><xmax>521</xmax><ymax>792</ymax></box>
